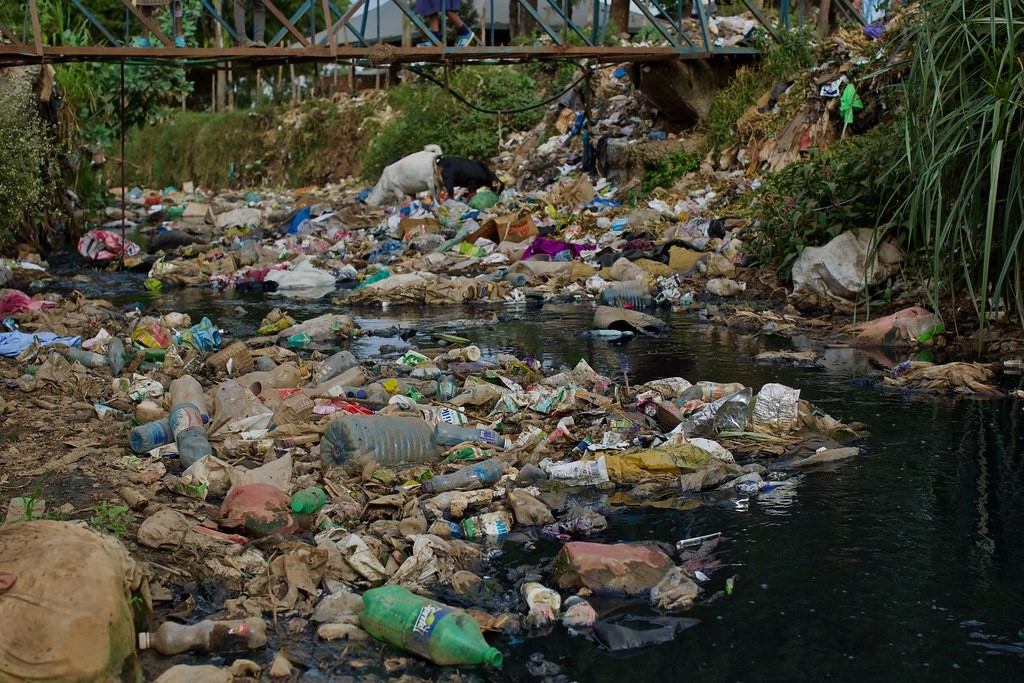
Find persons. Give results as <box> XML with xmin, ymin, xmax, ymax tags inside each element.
<box><xmin>234</xmin><ymin>0</ymin><xmax>267</xmax><ymax>48</ymax></box>
<box><xmin>131</xmin><ymin>0</ymin><xmax>186</xmax><ymax>48</ymax></box>
<box><xmin>415</xmin><ymin>0</ymin><xmax>474</xmax><ymax>47</ymax></box>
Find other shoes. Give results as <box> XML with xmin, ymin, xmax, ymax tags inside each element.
<box><xmin>239</xmin><ymin>39</ymin><xmax>257</xmax><ymax>47</ymax></box>
<box><xmin>257</xmin><ymin>40</ymin><xmax>267</xmax><ymax>47</ymax></box>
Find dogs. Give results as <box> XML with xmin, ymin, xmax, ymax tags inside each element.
<box><xmin>355</xmin><ymin>144</ymin><xmax>505</xmax><ymax>210</ymax></box>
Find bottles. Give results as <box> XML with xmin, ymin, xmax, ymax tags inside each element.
<box><xmin>358</xmin><ymin>584</ymin><xmax>502</xmax><ymax>667</ymax></box>
<box><xmin>138</xmin><ymin>617</ymin><xmax>267</xmax><ymax>655</ymax></box>
<box><xmin>63</xmin><ymin>258</ymin><xmax>747</xmax><ymax>533</ymax></box>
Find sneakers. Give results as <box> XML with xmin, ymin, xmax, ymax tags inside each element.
<box><xmin>175</xmin><ymin>36</ymin><xmax>186</xmax><ymax>48</ymax></box>
<box><xmin>132</xmin><ymin>37</ymin><xmax>150</xmax><ymax>48</ymax></box>
<box><xmin>416</xmin><ymin>39</ymin><xmax>435</xmax><ymax>47</ymax></box>
<box><xmin>457</xmin><ymin>30</ymin><xmax>475</xmax><ymax>47</ymax></box>
